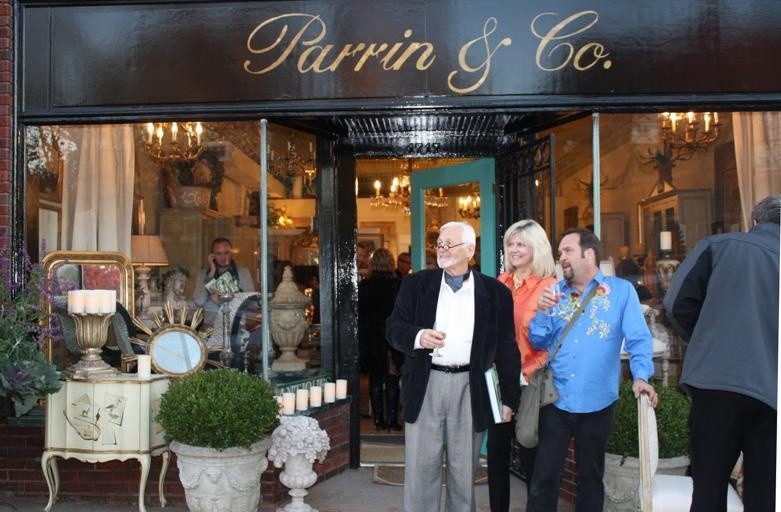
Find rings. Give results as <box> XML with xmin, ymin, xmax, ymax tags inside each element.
<box><xmin>655</xmin><ymin>392</ymin><xmax>662</xmax><ymax>400</ymax></box>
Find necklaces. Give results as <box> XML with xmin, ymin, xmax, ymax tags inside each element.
<box><xmin>512</xmin><ymin>270</ymin><xmax>533</xmax><ymax>289</ymax></box>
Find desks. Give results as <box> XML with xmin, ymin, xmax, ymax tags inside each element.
<box><xmin>40</xmin><ymin>372</ymin><xmax>169</xmax><ymax>512</ymax></box>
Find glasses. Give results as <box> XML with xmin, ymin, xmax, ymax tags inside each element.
<box><xmin>434</xmin><ymin>243</ymin><xmax>464</xmax><ymax>251</ymax></box>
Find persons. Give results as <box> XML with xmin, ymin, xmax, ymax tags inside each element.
<box><xmin>159</xmin><ymin>267</ymin><xmax>191</xmax><ymax>308</ymax></box>
<box><xmin>526</xmin><ymin>228</ymin><xmax>658</xmax><ymax>512</ymax></box>
<box><xmin>390</xmin><ymin>252</ymin><xmax>410</xmax><ymax>278</ymax></box>
<box><xmin>663</xmin><ymin>194</ymin><xmax>780</xmax><ymax>512</ymax></box>
<box><xmin>467</xmin><ymin>236</ymin><xmax>480</xmax><ymax>272</ymax></box>
<box><xmin>357</xmin><ymin>238</ymin><xmax>375</xmax><ymax>277</ymax></box>
<box><xmin>424</xmin><ymin>257</ymin><xmax>437</xmax><ymax>270</ymax></box>
<box><xmin>613</xmin><ymin>257</ymin><xmax>653</xmax><ymax>305</ymax></box>
<box><xmin>486</xmin><ymin>219</ymin><xmax>558</xmax><ymax>512</ymax></box>
<box><xmin>357</xmin><ymin>248</ymin><xmax>404</xmax><ymax>435</ymax></box>
<box><xmin>383</xmin><ymin>220</ymin><xmax>523</xmax><ymax>512</ymax></box>
<box><xmin>191</xmin><ymin>236</ymin><xmax>255</xmax><ymax>331</ymax></box>
<box><xmin>269</xmin><ymin>259</ymin><xmax>319</xmax><ymax>323</ymax></box>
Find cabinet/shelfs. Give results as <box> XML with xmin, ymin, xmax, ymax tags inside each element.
<box><xmin>637</xmin><ymin>185</ymin><xmax>715</xmax><ymax>261</ymax></box>
<box><xmin>586</xmin><ymin>208</ymin><xmax>627</xmax><ymax>260</ymax></box>
<box><xmin>162</xmin><ymin>208</ymin><xmax>235</xmax><ymax>288</ymax></box>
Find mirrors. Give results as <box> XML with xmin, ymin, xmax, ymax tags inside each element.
<box><xmin>124</xmin><ymin>301</ymin><xmax>212</xmax><ymax>377</ymax></box>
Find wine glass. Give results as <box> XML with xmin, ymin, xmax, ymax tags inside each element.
<box><xmin>430</xmin><ymin>324</ymin><xmax>446</xmax><ymax>357</ymax></box>
<box><xmin>548</xmin><ymin>284</ymin><xmax>560</xmax><ymax>317</ymax></box>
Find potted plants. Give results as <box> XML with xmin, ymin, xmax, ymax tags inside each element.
<box><xmin>153</xmin><ymin>363</ymin><xmax>277</xmax><ymax>511</ymax></box>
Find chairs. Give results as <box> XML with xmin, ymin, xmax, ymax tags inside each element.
<box><xmin>639</xmin><ymin>394</ymin><xmax>745</xmax><ymax>510</ymax></box>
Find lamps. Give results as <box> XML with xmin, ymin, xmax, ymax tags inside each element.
<box><xmin>457</xmin><ymin>190</ymin><xmax>483</xmax><ymax>220</ymax></box>
<box><xmin>660</xmin><ymin>111</ymin><xmax>726</xmax><ymax>151</ymax></box>
<box><xmin>64</xmin><ymin>289</ymin><xmax>118</xmax><ymax>381</ymax></box>
<box><xmin>143</xmin><ymin>124</ymin><xmax>207</xmax><ymax>163</ymax></box>
<box><xmin>370</xmin><ymin>164</ymin><xmax>450</xmax><ymax>210</ymax></box>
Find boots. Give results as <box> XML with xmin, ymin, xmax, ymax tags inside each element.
<box><xmin>369</xmin><ymin>373</ymin><xmax>387</xmax><ymax>433</ymax></box>
<box><xmin>385</xmin><ymin>374</ymin><xmax>401</xmax><ymax>436</ymax></box>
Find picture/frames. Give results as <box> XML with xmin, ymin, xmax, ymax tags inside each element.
<box><xmin>37</xmin><ymin>150</ymin><xmax>65</xmax><ymax>211</ymax></box>
<box><xmin>358</xmin><ymin>233</ymin><xmax>385</xmax><ymax>267</ymax></box>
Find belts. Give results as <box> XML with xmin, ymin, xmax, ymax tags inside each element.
<box><xmin>431</xmin><ymin>363</ymin><xmax>471</xmax><ymax>378</ymax></box>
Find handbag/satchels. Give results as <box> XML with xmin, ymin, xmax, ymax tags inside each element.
<box><xmin>516</xmin><ymin>285</ymin><xmax>600</xmax><ymax>449</ymax></box>
